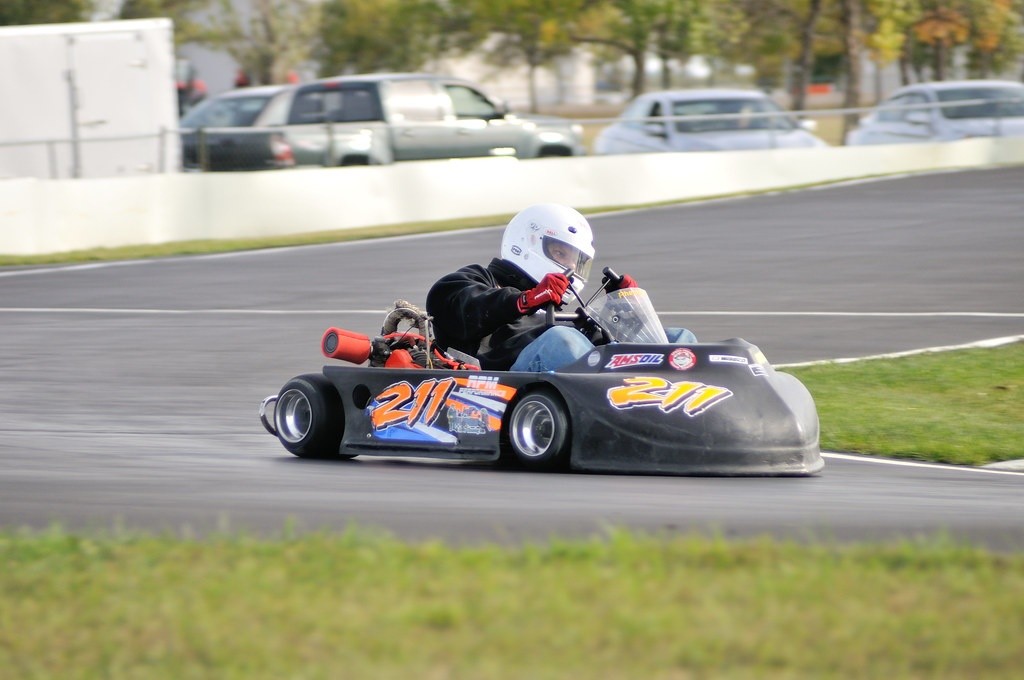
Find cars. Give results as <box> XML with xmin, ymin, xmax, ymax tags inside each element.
<box><xmin>846</xmin><ymin>80</ymin><xmax>1024</xmax><ymax>148</ymax></box>
<box><xmin>181</xmin><ymin>84</ymin><xmax>380</xmax><ymax>127</ymax></box>
<box><xmin>595</xmin><ymin>89</ymin><xmax>828</xmax><ymax>153</ymax></box>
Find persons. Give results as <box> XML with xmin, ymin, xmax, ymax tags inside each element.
<box><xmin>425</xmin><ymin>203</ymin><xmax>697</xmax><ymax>372</ymax></box>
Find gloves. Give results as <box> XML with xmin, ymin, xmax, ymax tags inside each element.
<box><xmin>601</xmin><ymin>273</ymin><xmax>638</xmax><ymax>294</ymax></box>
<box><xmin>517</xmin><ymin>272</ymin><xmax>574</xmax><ymax>317</ymax></box>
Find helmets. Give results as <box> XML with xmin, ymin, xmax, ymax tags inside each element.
<box><xmin>501</xmin><ymin>203</ymin><xmax>596</xmax><ymax>307</ymax></box>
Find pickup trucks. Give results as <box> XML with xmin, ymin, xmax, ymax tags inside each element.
<box><xmin>182</xmin><ymin>73</ymin><xmax>586</xmax><ymax>169</ymax></box>
